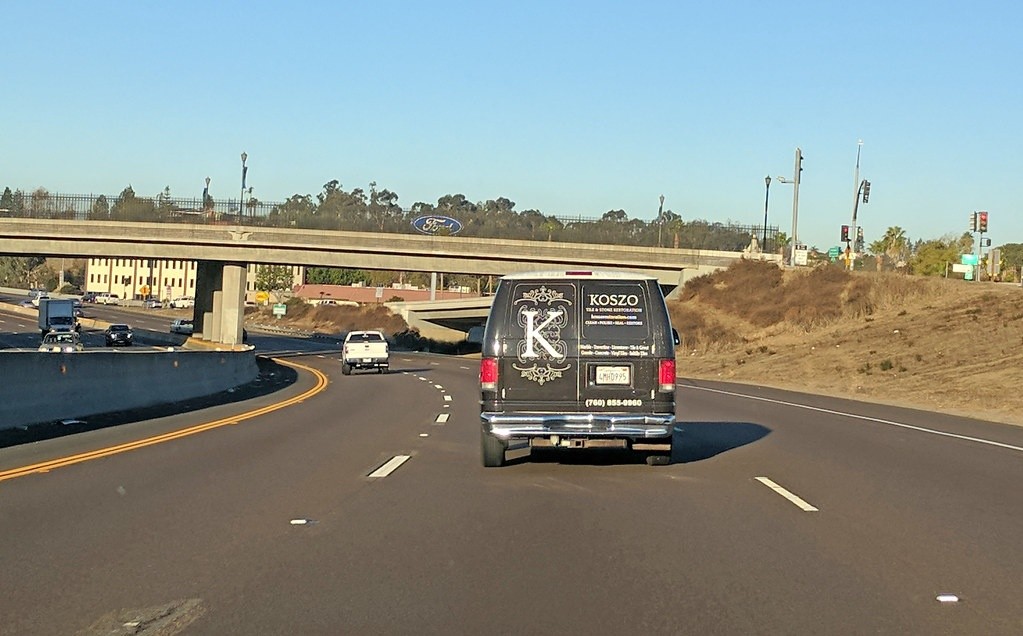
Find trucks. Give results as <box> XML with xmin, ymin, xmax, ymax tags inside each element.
<box><xmin>37</xmin><ymin>299</ymin><xmax>75</xmax><ymax>336</ymax></box>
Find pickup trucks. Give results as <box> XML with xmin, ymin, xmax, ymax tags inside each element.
<box><xmin>341</xmin><ymin>330</ymin><xmax>390</xmax><ymax>375</ymax></box>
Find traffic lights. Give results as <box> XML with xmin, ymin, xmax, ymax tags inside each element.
<box><xmin>969</xmin><ymin>211</ymin><xmax>977</xmax><ymax>232</ymax></box>
<box><xmin>863</xmin><ymin>180</ymin><xmax>870</xmax><ymax>203</ymax></box>
<box><xmin>979</xmin><ymin>211</ymin><xmax>987</xmax><ymax>232</ymax></box>
<box><xmin>841</xmin><ymin>225</ymin><xmax>848</xmax><ymax>242</ymax></box>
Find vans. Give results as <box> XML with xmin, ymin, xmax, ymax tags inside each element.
<box><xmin>467</xmin><ymin>269</ymin><xmax>681</xmax><ymax>467</ymax></box>
<box><xmin>28</xmin><ymin>288</ymin><xmax>47</xmax><ymax>297</ymax></box>
<box><xmin>318</xmin><ymin>300</ymin><xmax>338</xmax><ymax>307</ymax></box>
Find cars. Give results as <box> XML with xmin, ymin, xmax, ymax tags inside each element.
<box><xmin>81</xmin><ymin>294</ymin><xmax>96</xmax><ymax>303</ymax></box>
<box><xmin>244</xmin><ymin>301</ymin><xmax>256</xmax><ymax>307</ymax></box>
<box><xmin>175</xmin><ymin>319</ymin><xmax>193</xmax><ymax>336</ymax></box>
<box><xmin>74</xmin><ymin>308</ymin><xmax>84</xmax><ymax>318</ymax></box>
<box><xmin>783</xmin><ymin>257</ymin><xmax>790</xmax><ymax>265</ymax></box>
<box><xmin>37</xmin><ymin>329</ymin><xmax>84</xmax><ymax>352</ymax></box>
<box><xmin>243</xmin><ymin>328</ymin><xmax>248</xmax><ymax>344</ymax></box>
<box><xmin>18</xmin><ymin>300</ymin><xmax>36</xmax><ymax>309</ymax></box>
<box><xmin>32</xmin><ymin>295</ymin><xmax>51</xmax><ymax>309</ymax></box>
<box><xmin>94</xmin><ymin>293</ymin><xmax>119</xmax><ymax>305</ymax></box>
<box><xmin>813</xmin><ymin>260</ymin><xmax>829</xmax><ymax>267</ymax></box>
<box><xmin>989</xmin><ymin>274</ymin><xmax>1001</xmax><ymax>282</ymax></box>
<box><xmin>146</xmin><ymin>299</ymin><xmax>162</xmax><ymax>308</ymax></box>
<box><xmin>1020</xmin><ymin>258</ymin><xmax>1023</xmax><ymax>284</ymax></box>
<box><xmin>70</xmin><ymin>298</ymin><xmax>81</xmax><ymax>308</ymax></box>
<box><xmin>170</xmin><ymin>319</ymin><xmax>177</xmax><ymax>333</ymax></box>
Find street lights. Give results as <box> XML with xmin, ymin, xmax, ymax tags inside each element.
<box><xmin>762</xmin><ymin>174</ymin><xmax>771</xmax><ymax>252</ymax></box>
<box><xmin>205</xmin><ymin>177</ymin><xmax>210</xmax><ymax>224</ymax></box>
<box><xmin>658</xmin><ymin>194</ymin><xmax>665</xmax><ymax>248</ymax></box>
<box><xmin>239</xmin><ymin>152</ymin><xmax>248</xmax><ymax>226</ymax></box>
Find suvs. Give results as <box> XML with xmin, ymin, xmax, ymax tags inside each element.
<box><xmin>104</xmin><ymin>324</ymin><xmax>133</xmax><ymax>346</ymax></box>
<box><xmin>169</xmin><ymin>296</ymin><xmax>195</xmax><ymax>309</ymax></box>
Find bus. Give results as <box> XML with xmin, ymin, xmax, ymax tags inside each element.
<box><xmin>0</xmin><ymin>208</ymin><xmax>14</xmax><ymax>218</ymax></box>
